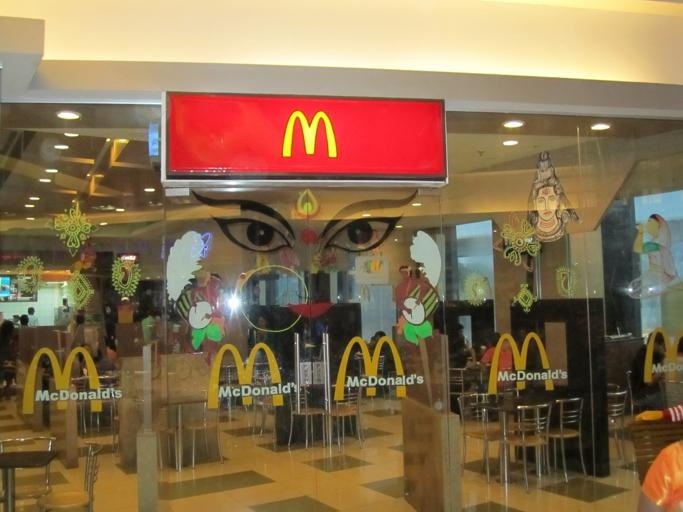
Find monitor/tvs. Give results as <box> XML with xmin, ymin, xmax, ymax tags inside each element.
<box><xmin>0</xmin><ymin>274</ymin><xmax>39</xmax><ymax>302</ymax></box>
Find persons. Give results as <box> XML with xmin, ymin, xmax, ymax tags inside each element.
<box><xmin>627</xmin><ymin>330</ymin><xmax>665</xmax><ymax>411</ymax></box>
<box><xmin>650</xmin><ymin>336</ymin><xmax>682</xmax><ymax>382</ymax></box>
<box><xmin>518</xmin><ymin>150</ymin><xmax>582</xmax><ymax>245</ymax></box>
<box><xmin>0</xmin><ymin>297</ymin><xmax>119</xmax><ymax>390</ymax></box>
<box><xmin>247</xmin><ymin>310</ymin><xmax>513</xmax><ymax>423</ymax></box>
<box><xmin>633</xmin><ymin>441</ymin><xmax>682</xmax><ymax>512</ymax></box>
<box><xmin>633</xmin><ymin>403</ymin><xmax>683</xmax><ymax>425</ymax></box>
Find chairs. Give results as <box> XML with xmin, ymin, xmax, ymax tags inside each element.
<box><xmin>2</xmin><ymin>431</ymin><xmax>104</xmax><ymax>510</ymax></box>
<box><xmin>2</xmin><ymin>325</ymin><xmax>534</xmax><ymax>475</ymax></box>
<box><xmin>456</xmin><ymin>381</ymin><xmax>630</xmax><ymax>496</ymax></box>
